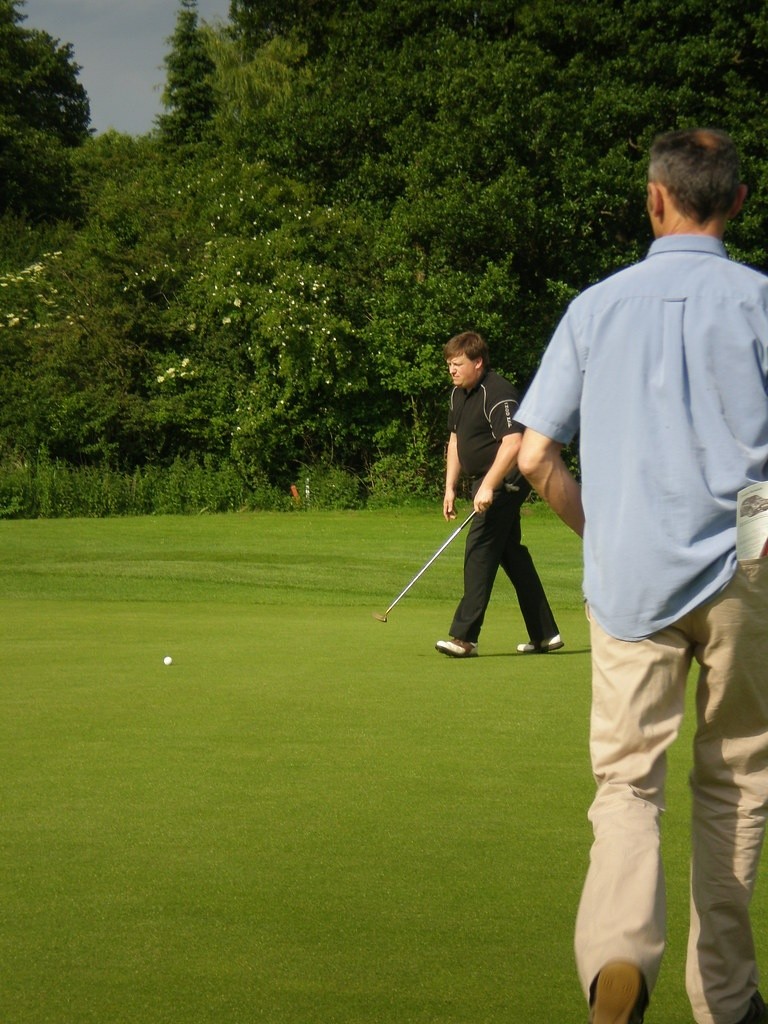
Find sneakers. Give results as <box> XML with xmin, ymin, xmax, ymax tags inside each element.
<box><xmin>517</xmin><ymin>633</ymin><xmax>563</xmax><ymax>653</ymax></box>
<box><xmin>435</xmin><ymin>639</ymin><xmax>478</xmax><ymax>657</ymax></box>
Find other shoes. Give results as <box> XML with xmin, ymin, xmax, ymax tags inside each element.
<box><xmin>589</xmin><ymin>962</ymin><xmax>649</xmax><ymax>1024</ymax></box>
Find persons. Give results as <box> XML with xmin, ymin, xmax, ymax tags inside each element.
<box><xmin>434</xmin><ymin>330</ymin><xmax>565</xmax><ymax>658</ymax></box>
<box><xmin>514</xmin><ymin>126</ymin><xmax>767</xmax><ymax>1024</ymax></box>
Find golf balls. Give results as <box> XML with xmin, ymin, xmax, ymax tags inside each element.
<box><xmin>164</xmin><ymin>657</ymin><xmax>173</xmax><ymax>665</ymax></box>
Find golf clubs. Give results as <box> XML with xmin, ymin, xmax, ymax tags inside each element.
<box><xmin>372</xmin><ymin>510</ymin><xmax>476</xmax><ymax>622</ymax></box>
